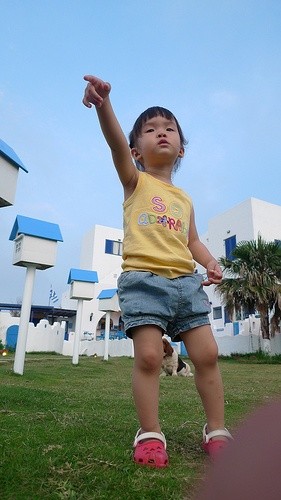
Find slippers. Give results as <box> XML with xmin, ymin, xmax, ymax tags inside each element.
<box><xmin>202</xmin><ymin>423</ymin><xmax>234</xmax><ymax>457</ymax></box>
<box><xmin>133</xmin><ymin>428</ymin><xmax>169</xmax><ymax>468</ymax></box>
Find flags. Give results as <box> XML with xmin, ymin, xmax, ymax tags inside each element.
<box><xmin>49</xmin><ymin>290</ymin><xmax>59</xmax><ymax>303</ymax></box>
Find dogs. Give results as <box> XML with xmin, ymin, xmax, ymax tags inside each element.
<box><xmin>159</xmin><ymin>337</ymin><xmax>195</xmax><ymax>377</ymax></box>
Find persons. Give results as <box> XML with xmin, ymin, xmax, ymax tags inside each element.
<box><xmin>82</xmin><ymin>75</ymin><xmax>234</xmax><ymax>468</ymax></box>
